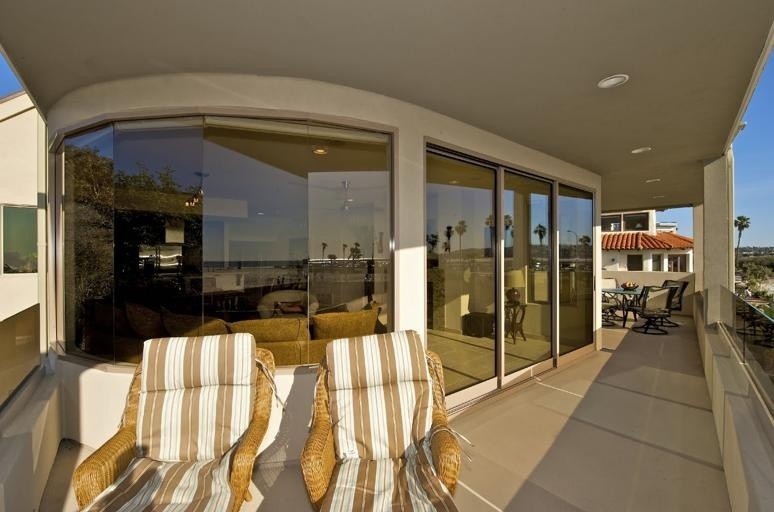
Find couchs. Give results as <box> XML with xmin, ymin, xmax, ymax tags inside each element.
<box><xmin>113</xmin><ymin>300</ymin><xmax>377</xmax><ymax>367</ymax></box>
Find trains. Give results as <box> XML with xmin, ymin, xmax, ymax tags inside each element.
<box><xmin>307</xmin><ymin>257</ymin><xmax>391</xmax><ymax>269</ymax></box>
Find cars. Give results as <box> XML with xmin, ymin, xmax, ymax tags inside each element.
<box><xmin>155</xmin><ymin>274</ymin><xmax>185</xmax><ymax>293</ymax></box>
<box><xmin>528</xmin><ymin>262</ymin><xmax>593</xmax><ymax>272</ymax></box>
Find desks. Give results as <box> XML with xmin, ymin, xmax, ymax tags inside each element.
<box><xmin>505</xmin><ymin>303</ymin><xmax>527</xmax><ymax>344</ymax></box>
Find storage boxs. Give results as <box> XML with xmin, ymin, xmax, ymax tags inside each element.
<box><xmin>462</xmin><ymin>312</ymin><xmax>494</xmax><ymax>336</ymax></box>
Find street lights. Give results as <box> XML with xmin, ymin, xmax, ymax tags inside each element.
<box><xmin>567</xmin><ymin>230</ymin><xmax>579</xmax><ymax>263</ymax></box>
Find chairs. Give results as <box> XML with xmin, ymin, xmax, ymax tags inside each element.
<box><xmin>71</xmin><ymin>331</ymin><xmax>276</xmax><ymax>512</ymax></box>
<box><xmin>257</xmin><ymin>290</ymin><xmax>318</xmax><ymax>317</ymax></box>
<box><xmin>299</xmin><ymin>330</ymin><xmax>458</xmax><ymax>511</ymax></box>
<box><xmin>600</xmin><ymin>280</ymin><xmax>688</xmax><ymax>336</ymax></box>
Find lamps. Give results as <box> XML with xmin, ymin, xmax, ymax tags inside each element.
<box><xmin>183</xmin><ymin>172</ymin><xmax>210</xmax><ymax>208</ymax></box>
<box><xmin>341</xmin><ymin>179</ymin><xmax>351</xmax><ymax>212</ymax></box>
<box><xmin>504</xmin><ymin>271</ymin><xmax>524</xmax><ymax>304</ymax></box>
